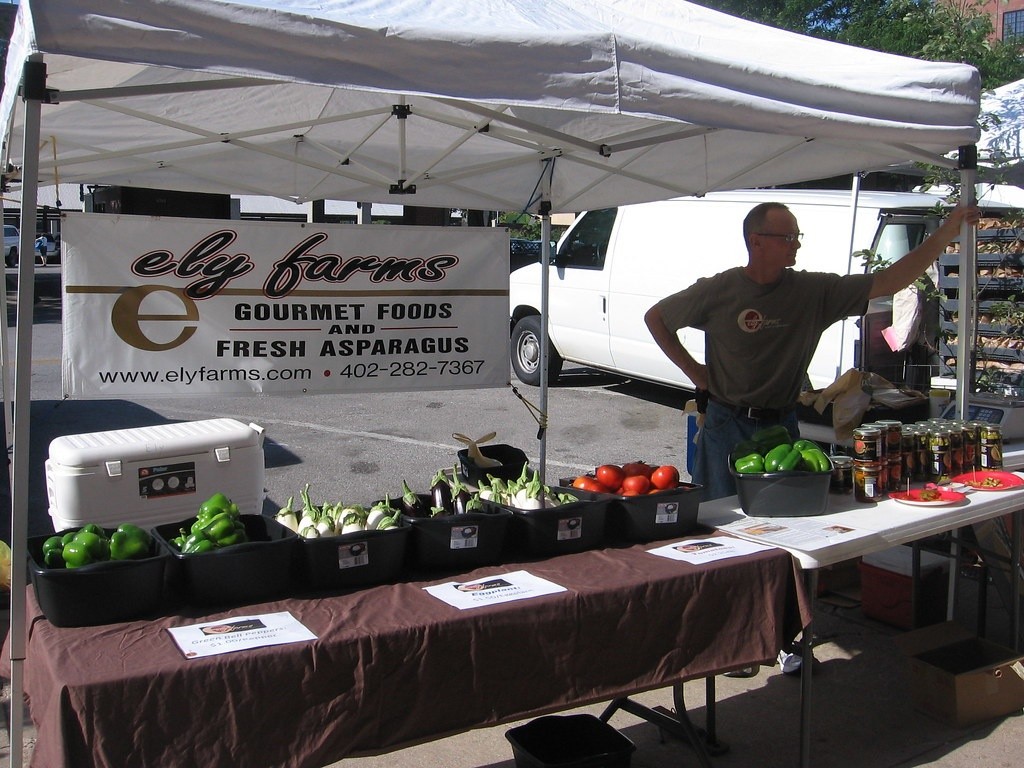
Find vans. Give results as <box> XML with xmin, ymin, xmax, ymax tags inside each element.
<box><xmin>510</xmin><ymin>189</ymin><xmax>1024</xmax><ymax>398</ymax></box>
<box><xmin>3</xmin><ymin>225</ymin><xmax>19</xmax><ymax>267</ymax></box>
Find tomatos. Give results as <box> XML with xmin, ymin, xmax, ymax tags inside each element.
<box><xmin>572</xmin><ymin>460</ymin><xmax>680</xmax><ymax>497</ymax></box>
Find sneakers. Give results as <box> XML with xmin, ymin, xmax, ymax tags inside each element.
<box><xmin>724</xmin><ymin>665</ymin><xmax>752</xmax><ymax>678</ymax></box>
<box><xmin>774</xmin><ymin>645</ymin><xmax>819</xmax><ymax>675</ymax></box>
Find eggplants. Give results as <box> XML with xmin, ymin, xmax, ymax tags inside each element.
<box><xmin>280</xmin><ymin>459</ymin><xmax>582</xmax><ymax>541</ymax></box>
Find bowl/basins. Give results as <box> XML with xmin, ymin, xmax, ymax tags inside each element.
<box><xmin>727</xmin><ymin>453</ymin><xmax>834</xmax><ymax>517</ymax></box>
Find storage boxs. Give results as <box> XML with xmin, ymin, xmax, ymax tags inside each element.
<box><xmin>858</xmin><ymin>551</ymin><xmax>950</xmax><ymax>630</ymax></box>
<box><xmin>44</xmin><ymin>417</ymin><xmax>265</xmax><ymax>535</ymax></box>
<box><xmin>727</xmin><ymin>439</ymin><xmax>834</xmax><ymax>517</ymax></box>
<box><xmin>28</xmin><ymin>471</ymin><xmax>704</xmax><ymax>631</ymax></box>
<box><xmin>503</xmin><ymin>714</ymin><xmax>635</xmax><ymax>767</ymax></box>
<box><xmin>457</xmin><ymin>443</ymin><xmax>531</xmax><ymax>488</ymax></box>
<box><xmin>895</xmin><ymin>620</ymin><xmax>1024</xmax><ymax>728</ymax></box>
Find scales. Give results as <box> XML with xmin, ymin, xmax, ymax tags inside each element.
<box><xmin>939</xmin><ymin>392</ymin><xmax>1024</xmax><ymax>444</ymax></box>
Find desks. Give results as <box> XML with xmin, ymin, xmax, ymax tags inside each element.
<box><xmin>1</xmin><ymin>515</ymin><xmax>812</xmax><ymax>767</ymax></box>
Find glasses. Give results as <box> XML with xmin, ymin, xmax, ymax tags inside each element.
<box><xmin>757</xmin><ymin>233</ymin><xmax>804</xmax><ymax>242</ymax></box>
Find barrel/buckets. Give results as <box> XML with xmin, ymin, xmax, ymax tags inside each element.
<box><xmin>928</xmin><ymin>389</ymin><xmax>951</xmax><ymax>419</ymax></box>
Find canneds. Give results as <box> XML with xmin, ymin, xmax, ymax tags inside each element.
<box><xmin>832</xmin><ymin>419</ymin><xmax>1002</xmax><ymax>503</ymax></box>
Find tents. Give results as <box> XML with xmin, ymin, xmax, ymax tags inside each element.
<box><xmin>0</xmin><ymin>0</ymin><xmax>982</xmax><ymax>768</ymax></box>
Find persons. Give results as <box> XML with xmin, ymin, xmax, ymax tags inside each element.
<box><xmin>38</xmin><ymin>232</ymin><xmax>48</xmax><ymax>267</ymax></box>
<box><xmin>644</xmin><ymin>198</ymin><xmax>980</xmax><ymax>676</ymax></box>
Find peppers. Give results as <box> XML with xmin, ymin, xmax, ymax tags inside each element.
<box><xmin>167</xmin><ymin>493</ymin><xmax>249</xmax><ymax>554</ymax></box>
<box><xmin>43</xmin><ymin>524</ymin><xmax>155</xmax><ymax>572</ymax></box>
<box><xmin>734</xmin><ymin>425</ymin><xmax>830</xmax><ymax>472</ymax></box>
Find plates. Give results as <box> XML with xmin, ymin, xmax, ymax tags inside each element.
<box><xmin>887</xmin><ymin>488</ymin><xmax>966</xmax><ymax>507</ymax></box>
<box><xmin>952</xmin><ymin>471</ymin><xmax>1024</xmax><ymax>491</ymax></box>
